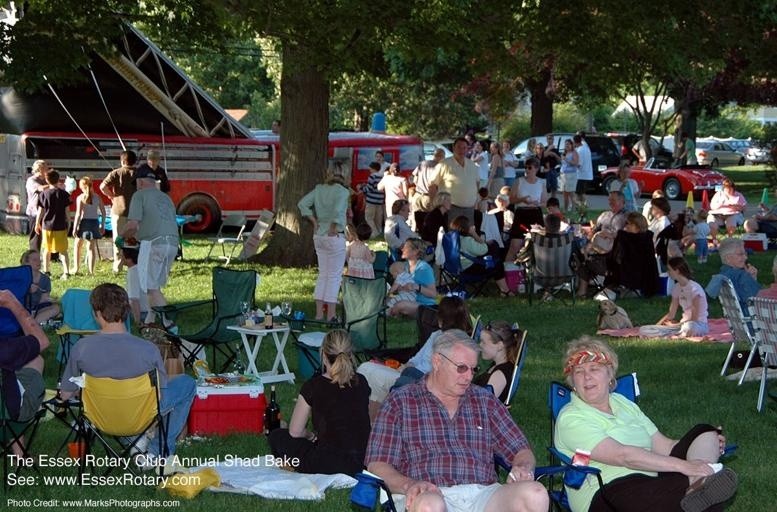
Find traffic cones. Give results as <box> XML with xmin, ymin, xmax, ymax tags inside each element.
<box><xmin>700</xmin><ymin>188</ymin><xmax>711</xmax><ymax>210</ymax></box>
<box><xmin>759</xmin><ymin>188</ymin><xmax>769</xmax><ymax>208</ymax></box>
<box><xmin>685</xmin><ymin>191</ymin><xmax>694</xmax><ymax>210</ymax></box>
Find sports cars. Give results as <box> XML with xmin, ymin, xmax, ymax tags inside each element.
<box><xmin>603</xmin><ymin>155</ymin><xmax>731</xmax><ymax>198</ymax></box>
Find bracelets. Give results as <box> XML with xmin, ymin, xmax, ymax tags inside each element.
<box><xmin>308</xmin><ymin>434</ymin><xmax>318</xmax><ymax>443</ymax></box>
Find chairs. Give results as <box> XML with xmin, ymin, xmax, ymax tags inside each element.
<box><xmin>362</xmin><ymin>311</ymin><xmax>485</xmax><ymax>366</ymax></box>
<box><xmin>42</xmin><ymin>329</ymin><xmax>110</xmax><ymax>460</ymax></box>
<box><xmin>288</xmin><ymin>274</ymin><xmax>388</xmax><ymax>380</ymax></box>
<box><xmin>77</xmin><ymin>367</ymin><xmax>174</xmax><ymax>485</ymax></box>
<box><xmin>547</xmin><ymin>370</ymin><xmax>739</xmax><ymax>510</ymax></box>
<box><xmin>712</xmin><ymin>274</ymin><xmax>758</xmax><ymax>384</ymax></box>
<box><xmin>56</xmin><ymin>287</ymin><xmax>132</xmax><ymax>384</ymax></box>
<box><xmin>716</xmin><ymin>208</ymin><xmax>744</xmax><ymax>241</ymax></box>
<box><xmin>390</xmin><ymin>204</ymin><xmax>686</xmax><ymax>309</ymax></box>
<box><xmin>0</xmin><ymin>368</ymin><xmax>45</xmax><ymax>490</ymax></box>
<box><xmin>745</xmin><ymin>296</ymin><xmax>776</xmax><ymax>411</ymax></box>
<box><xmin>502</xmin><ymin>322</ymin><xmax>529</xmax><ymax>410</ymax></box>
<box><xmin>150</xmin><ymin>266</ymin><xmax>259</xmax><ymax>373</ymax></box>
<box><xmin>205</xmin><ymin>213</ymin><xmax>247</xmax><ymax>267</ymax></box>
<box><xmin>0</xmin><ymin>264</ymin><xmax>34</xmax><ymax>338</ymax></box>
<box><xmin>352</xmin><ymin>386</ymin><xmax>564</xmax><ymax>512</ymax></box>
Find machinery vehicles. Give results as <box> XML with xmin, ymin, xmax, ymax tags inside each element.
<box><xmin>0</xmin><ymin>2</ymin><xmax>425</xmax><ymax>234</ymax></box>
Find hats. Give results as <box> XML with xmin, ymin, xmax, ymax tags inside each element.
<box><xmin>130</xmin><ymin>167</ymin><xmax>156</xmax><ymax>186</ymax></box>
<box><xmin>590</xmin><ymin>229</ymin><xmax>614</xmax><ymax>255</ymax></box>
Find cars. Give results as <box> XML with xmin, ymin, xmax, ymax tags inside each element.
<box><xmin>654</xmin><ymin>134</ymin><xmax>769</xmax><ymax>166</ymax></box>
<box><xmin>424</xmin><ymin>144</ymin><xmax>454</xmax><ymax>163</ymax></box>
<box><xmin>512</xmin><ymin>136</ymin><xmax>614</xmax><ymax>187</ymax></box>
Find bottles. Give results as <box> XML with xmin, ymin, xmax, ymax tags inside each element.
<box><xmin>232</xmin><ymin>342</ymin><xmax>244</xmax><ymax>375</ymax></box>
<box><xmin>263</xmin><ymin>300</ymin><xmax>274</xmax><ymax>330</ymax></box>
<box><xmin>263</xmin><ymin>384</ymin><xmax>280</xmax><ymax>436</ymax></box>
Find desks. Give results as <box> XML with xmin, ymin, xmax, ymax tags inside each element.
<box><xmin>225</xmin><ymin>322</ymin><xmax>296</xmax><ymax>384</ymax></box>
<box><xmin>94</xmin><ymin>213</ymin><xmax>202</xmax><ymax>266</ymax></box>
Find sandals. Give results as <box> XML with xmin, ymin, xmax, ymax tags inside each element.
<box><xmin>499</xmin><ymin>289</ymin><xmax>516</xmax><ymax>299</ymax></box>
<box><xmin>574</xmin><ymin>292</ymin><xmax>586</xmax><ymax>300</ymax></box>
<box><xmin>679</xmin><ymin>467</ymin><xmax>737</xmax><ymax>512</ymax></box>
<box><xmin>325</xmin><ymin>314</ymin><xmax>342</xmax><ymax>324</ymax></box>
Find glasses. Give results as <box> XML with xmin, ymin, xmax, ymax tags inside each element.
<box><xmin>730</xmin><ymin>251</ymin><xmax>749</xmax><ymax>257</ymax></box>
<box><xmin>484</xmin><ymin>324</ymin><xmax>504</xmax><ymax>341</ymax></box>
<box><xmin>438</xmin><ymin>352</ymin><xmax>480</xmax><ymax>375</ymax></box>
<box><xmin>523</xmin><ymin>165</ymin><xmax>532</xmax><ymax>170</ymax></box>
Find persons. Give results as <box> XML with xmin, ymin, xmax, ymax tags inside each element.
<box><xmin>0</xmin><ymin>288</ymin><xmax>54</xmax><ymax>465</ymax></box>
<box><xmin>363</xmin><ymin>329</ymin><xmax>554</xmax><ymax>512</ymax></box>
<box><xmin>1</xmin><ymin>128</ymin><xmax>776</xmax><ymax>372</ymax></box>
<box><xmin>56</xmin><ymin>282</ymin><xmax>198</xmax><ymax>476</ymax></box>
<box><xmin>471</xmin><ymin>316</ymin><xmax>527</xmax><ymax>405</ymax></box>
<box><xmin>267</xmin><ymin>326</ymin><xmax>372</xmax><ymax>476</ymax></box>
<box><xmin>554</xmin><ymin>337</ymin><xmax>738</xmax><ymax>510</ymax></box>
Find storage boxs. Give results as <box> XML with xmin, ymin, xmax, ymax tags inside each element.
<box><xmin>188</xmin><ymin>372</ymin><xmax>266</xmax><ymax>432</ymax></box>
<box><xmin>743</xmin><ymin>233</ymin><xmax>768</xmax><ymax>250</ymax></box>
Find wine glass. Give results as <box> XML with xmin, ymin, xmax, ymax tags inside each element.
<box><xmin>281</xmin><ymin>301</ymin><xmax>293</xmax><ymax>326</ymax></box>
<box><xmin>238</xmin><ymin>299</ymin><xmax>251</xmax><ymax>326</ymax></box>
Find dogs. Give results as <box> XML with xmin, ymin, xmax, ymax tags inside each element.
<box><xmin>595</xmin><ymin>300</ymin><xmax>634</xmax><ymax>330</ymax></box>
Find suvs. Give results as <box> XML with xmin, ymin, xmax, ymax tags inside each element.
<box><xmin>603</xmin><ymin>132</ymin><xmax>673</xmax><ymax>164</ymax></box>
